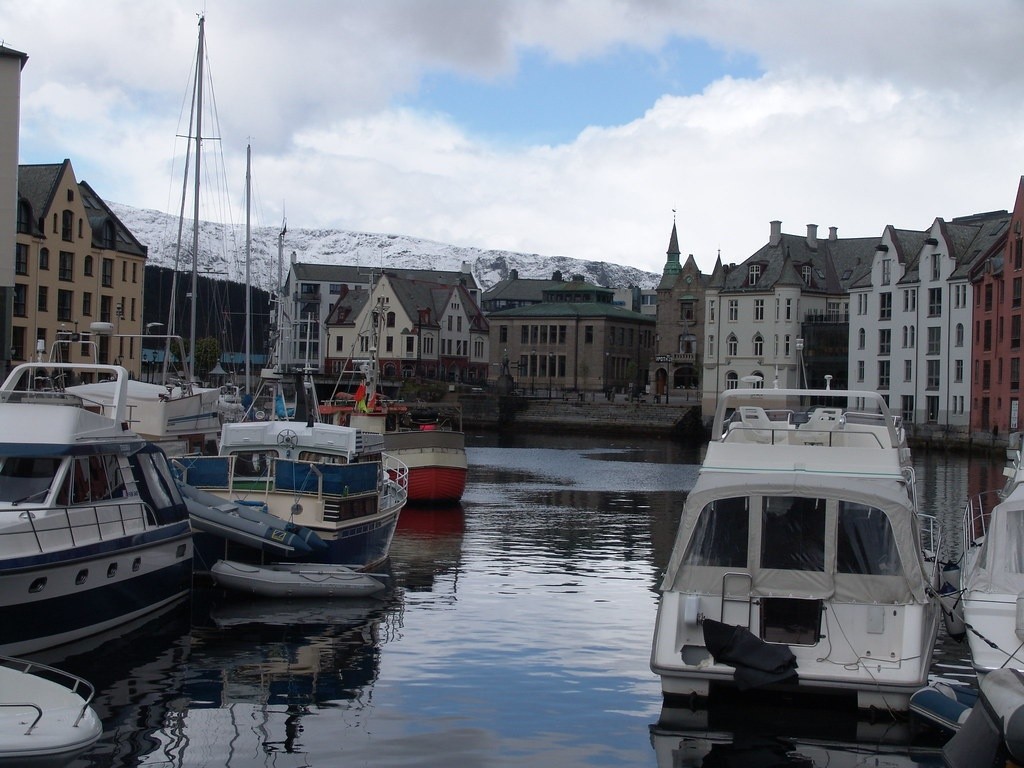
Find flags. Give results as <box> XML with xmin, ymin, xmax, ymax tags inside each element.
<box><xmin>353</xmin><ymin>385</ymin><xmax>367</xmax><ymax>402</ymax></box>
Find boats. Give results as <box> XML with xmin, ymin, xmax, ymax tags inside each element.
<box><xmin>320</xmin><ymin>295</ymin><xmax>468</xmax><ymax>507</ymax></box>
<box><xmin>649</xmin><ymin>373</ymin><xmax>945</xmax><ymax>708</ymax></box>
<box><xmin>212</xmin><ymin>558</ymin><xmax>391</xmax><ymax>598</ymax></box>
<box><xmin>177</xmin><ymin>311</ymin><xmax>408</xmax><ymax>571</ymax></box>
<box><xmin>210</xmin><ymin>590</ymin><xmax>384</xmax><ymax>627</ymax></box>
<box><xmin>958</xmin><ymin>451</ymin><xmax>1024</xmax><ymax>768</ymax></box>
<box><xmin>650</xmin><ymin>697</ymin><xmax>928</xmax><ymax>768</ymax></box>
<box><xmin>0</xmin><ymin>361</ymin><xmax>196</xmax><ymax>634</ymax></box>
<box><xmin>0</xmin><ymin>570</ymin><xmax>195</xmax><ymax>668</ymax></box>
<box><xmin>1</xmin><ymin>657</ymin><xmax>103</xmax><ymax>768</ymax></box>
<box><xmin>65</xmin><ymin>14</ymin><xmax>321</xmax><ymax>456</ymax></box>
<box><xmin>174</xmin><ymin>477</ymin><xmax>329</xmax><ymax>559</ymax></box>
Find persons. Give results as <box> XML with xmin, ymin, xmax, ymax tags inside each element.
<box><xmin>86</xmin><ymin>455</ymin><xmax>108</xmax><ymax>499</ymax></box>
<box><xmin>2</xmin><ymin>457</ymin><xmax>32</xmax><ymax>478</ymax></box>
<box><xmin>501</xmin><ymin>353</ymin><xmax>511</xmax><ymax>377</ymax></box>
